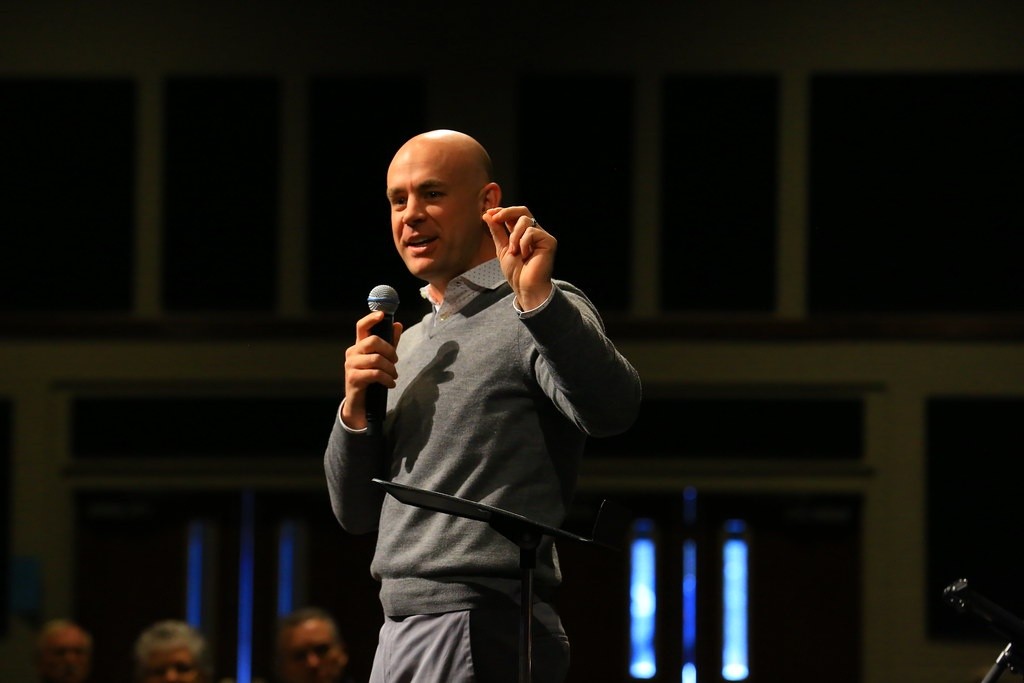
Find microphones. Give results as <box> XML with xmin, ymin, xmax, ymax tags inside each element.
<box><xmin>365</xmin><ymin>285</ymin><xmax>400</xmax><ymax>436</ymax></box>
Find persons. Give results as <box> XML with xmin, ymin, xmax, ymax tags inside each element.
<box><xmin>135</xmin><ymin>619</ymin><xmax>215</xmax><ymax>683</ymax></box>
<box><xmin>276</xmin><ymin>606</ymin><xmax>349</xmax><ymax>683</ymax></box>
<box><xmin>323</xmin><ymin>128</ymin><xmax>643</xmax><ymax>683</ymax></box>
<box><xmin>36</xmin><ymin>620</ymin><xmax>93</xmax><ymax>683</ymax></box>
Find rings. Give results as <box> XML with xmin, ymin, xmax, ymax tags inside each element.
<box><xmin>532</xmin><ymin>219</ymin><xmax>536</xmax><ymax>227</ymax></box>
